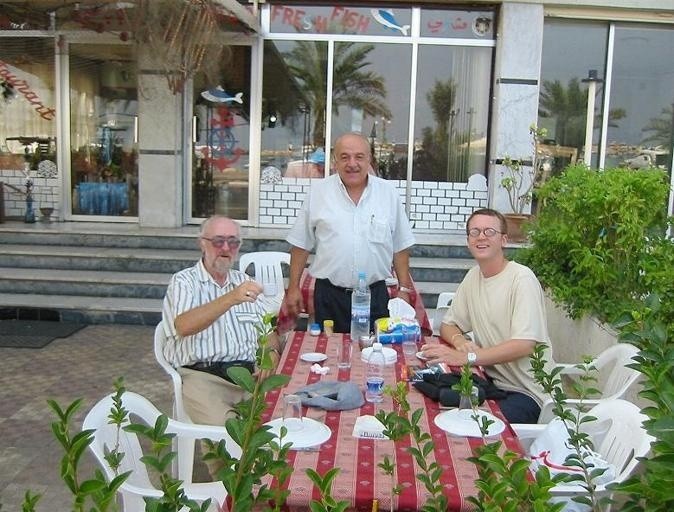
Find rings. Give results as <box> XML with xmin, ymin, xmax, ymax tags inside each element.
<box><xmin>246</xmin><ymin>291</ymin><xmax>250</xmax><ymax>296</ymax></box>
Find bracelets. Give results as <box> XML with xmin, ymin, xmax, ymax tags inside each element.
<box><xmin>398</xmin><ymin>285</ymin><xmax>413</xmax><ymax>296</ymax></box>
<box><xmin>270</xmin><ymin>348</ymin><xmax>281</xmax><ymax>358</ymax></box>
<box><xmin>450</xmin><ymin>334</ymin><xmax>464</xmax><ymax>344</ymax></box>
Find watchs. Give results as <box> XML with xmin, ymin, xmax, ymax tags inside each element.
<box><xmin>468</xmin><ymin>351</ymin><xmax>477</xmax><ymax>367</ymax></box>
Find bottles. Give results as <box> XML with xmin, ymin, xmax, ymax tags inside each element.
<box><xmin>324</xmin><ymin>320</ymin><xmax>333</xmax><ymax>337</ymax></box>
<box><xmin>310</xmin><ymin>323</ymin><xmax>322</xmax><ymax>336</ymax></box>
<box><xmin>365</xmin><ymin>343</ymin><xmax>385</xmax><ymax>403</ymax></box>
<box><xmin>350</xmin><ymin>271</ymin><xmax>371</xmax><ymax>341</ymax></box>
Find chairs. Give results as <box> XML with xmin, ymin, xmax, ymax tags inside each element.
<box><xmin>239</xmin><ymin>250</ymin><xmax>291</xmax><ymax>320</ymax></box>
<box><xmin>509</xmin><ymin>400</ymin><xmax>661</xmax><ymax>510</ymax></box>
<box><xmin>532</xmin><ymin>143</ymin><xmax>577</xmax><ymax>219</ymax></box>
<box><xmin>426</xmin><ymin>291</ymin><xmax>457</xmax><ymax>333</ymax></box>
<box><xmin>537</xmin><ymin>343</ymin><xmax>646</xmax><ymax>421</ymax></box>
<box><xmin>154</xmin><ymin>314</ymin><xmax>195</xmax><ymax>426</ymax></box>
<box><xmin>83</xmin><ymin>390</ymin><xmax>240</xmax><ymax>512</ymax></box>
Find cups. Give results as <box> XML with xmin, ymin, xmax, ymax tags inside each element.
<box><xmin>402</xmin><ymin>325</ymin><xmax>416</xmax><ymax>353</ymax></box>
<box><xmin>282</xmin><ymin>394</ymin><xmax>305</xmax><ymax>432</ymax></box>
<box><xmin>336</xmin><ymin>343</ymin><xmax>352</xmax><ymax>368</ymax></box>
<box><xmin>261</xmin><ymin>284</ymin><xmax>279</xmax><ymax>297</ymax></box>
<box><xmin>383</xmin><ymin>277</ymin><xmax>399</xmax><ymax>300</ymax></box>
<box><xmin>458</xmin><ymin>386</ymin><xmax>480</xmax><ymax>420</ymax></box>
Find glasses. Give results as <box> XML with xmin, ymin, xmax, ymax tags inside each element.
<box><xmin>468</xmin><ymin>227</ymin><xmax>504</xmax><ymax>237</ymax></box>
<box><xmin>201</xmin><ymin>235</ymin><xmax>241</xmax><ymax>250</ymax></box>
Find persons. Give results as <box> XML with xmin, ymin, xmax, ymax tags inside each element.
<box><xmin>285</xmin><ymin>132</ymin><xmax>415</xmax><ymax>336</ymax></box>
<box><xmin>160</xmin><ymin>213</ymin><xmax>282</xmax><ymax>485</ymax></box>
<box><xmin>420</xmin><ymin>208</ymin><xmax>563</xmax><ymax>424</ymax></box>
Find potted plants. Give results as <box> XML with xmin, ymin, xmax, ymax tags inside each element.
<box><xmin>498</xmin><ymin>123</ymin><xmax>554</xmax><ymax>245</ymax></box>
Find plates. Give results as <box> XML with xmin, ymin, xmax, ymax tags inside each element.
<box><xmin>433</xmin><ymin>409</ymin><xmax>506</xmax><ymax>438</ymax></box>
<box><xmin>299</xmin><ymin>352</ymin><xmax>328</xmax><ymax>362</ymax></box>
<box><xmin>415</xmin><ymin>351</ymin><xmax>431</xmax><ymax>360</ymax></box>
<box><xmin>260</xmin><ymin>416</ymin><xmax>332</xmax><ymax>448</ymax></box>
